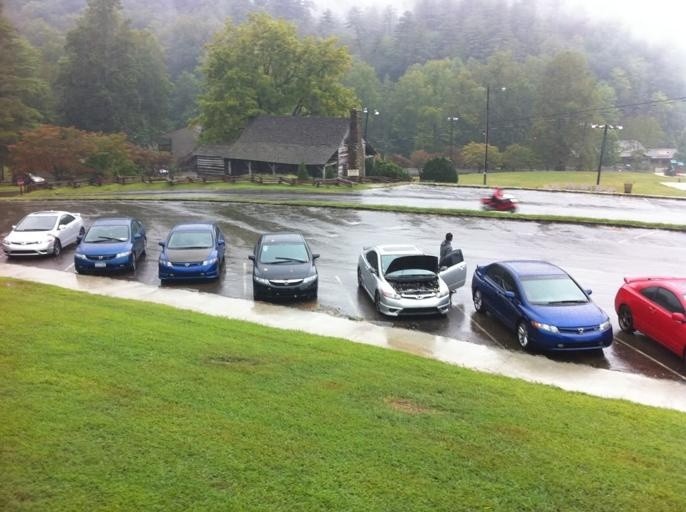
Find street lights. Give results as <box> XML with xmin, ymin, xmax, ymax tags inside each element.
<box><xmin>363</xmin><ymin>105</ymin><xmax>380</xmax><ymax>142</ymax></box>
<box><xmin>591</xmin><ymin>123</ymin><xmax>624</xmax><ymax>185</ymax></box>
<box><xmin>483</xmin><ymin>83</ymin><xmax>507</xmax><ymax>185</ymax></box>
<box><xmin>446</xmin><ymin>113</ymin><xmax>460</xmax><ymax>159</ymax></box>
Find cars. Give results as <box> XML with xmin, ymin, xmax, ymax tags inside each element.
<box><xmin>248</xmin><ymin>230</ymin><xmax>321</xmax><ymax>300</ymax></box>
<box><xmin>3</xmin><ymin>210</ymin><xmax>88</xmax><ymax>261</ymax></box>
<box><xmin>25</xmin><ymin>173</ymin><xmax>44</xmax><ymax>185</ymax></box>
<box><xmin>472</xmin><ymin>259</ymin><xmax>613</xmax><ymax>354</ymax></box>
<box><xmin>157</xmin><ymin>223</ymin><xmax>227</xmax><ymax>285</ymax></box>
<box><xmin>360</xmin><ymin>246</ymin><xmax>468</xmax><ymax>319</ymax></box>
<box><xmin>74</xmin><ymin>217</ymin><xmax>147</xmax><ymax>275</ymax></box>
<box><xmin>614</xmin><ymin>276</ymin><xmax>685</xmax><ymax>357</ymax></box>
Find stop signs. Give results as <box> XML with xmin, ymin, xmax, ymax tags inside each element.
<box><xmin>16</xmin><ymin>178</ymin><xmax>24</xmax><ymax>186</ymax></box>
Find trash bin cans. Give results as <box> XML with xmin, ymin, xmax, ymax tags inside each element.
<box><xmin>624</xmin><ymin>182</ymin><xmax>632</xmax><ymax>193</ymax></box>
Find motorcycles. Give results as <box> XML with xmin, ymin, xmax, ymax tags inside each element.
<box><xmin>477</xmin><ymin>194</ymin><xmax>517</xmax><ymax>214</ymax></box>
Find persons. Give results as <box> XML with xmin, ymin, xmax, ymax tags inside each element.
<box><xmin>490</xmin><ymin>187</ymin><xmax>503</xmax><ymax>204</ymax></box>
<box><xmin>439</xmin><ymin>231</ymin><xmax>457</xmax><ymax>294</ymax></box>
